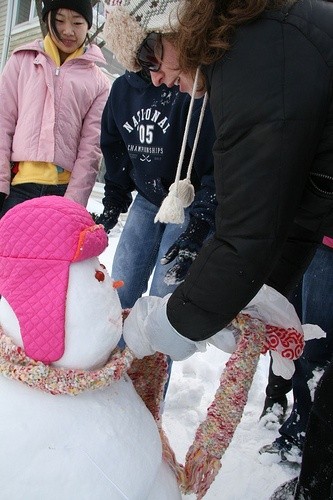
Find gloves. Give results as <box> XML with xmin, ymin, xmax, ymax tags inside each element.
<box><xmin>159</xmin><ymin>216</ymin><xmax>213</xmax><ymax>286</ymax></box>
<box><xmin>122</xmin><ymin>293</ymin><xmax>209</xmax><ymax>362</ymax></box>
<box><xmin>95</xmin><ymin>204</ymin><xmax>122</xmax><ymax>234</ymax></box>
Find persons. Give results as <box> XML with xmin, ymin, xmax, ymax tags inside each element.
<box><xmin>259</xmin><ymin>233</ymin><xmax>333</xmax><ymax>466</ymax></box>
<box><xmin>100</xmin><ymin>72</ymin><xmax>216</xmax><ymax>399</ymax></box>
<box><xmin>0</xmin><ymin>0</ymin><xmax>110</xmax><ymax>219</ymax></box>
<box><xmin>104</xmin><ymin>0</ymin><xmax>333</xmax><ymax>500</ymax></box>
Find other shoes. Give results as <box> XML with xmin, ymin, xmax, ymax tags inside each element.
<box><xmin>258</xmin><ymin>435</ymin><xmax>304</xmax><ymax>466</ymax></box>
<box><xmin>269</xmin><ymin>476</ymin><xmax>313</xmax><ymax>500</ymax></box>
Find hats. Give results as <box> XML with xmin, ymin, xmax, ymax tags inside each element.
<box><xmin>103</xmin><ymin>0</ymin><xmax>190</xmax><ymax>74</ymax></box>
<box><xmin>41</xmin><ymin>0</ymin><xmax>93</xmax><ymax>30</ymax></box>
<box><xmin>0</xmin><ymin>195</ymin><xmax>109</xmax><ymax>363</ymax></box>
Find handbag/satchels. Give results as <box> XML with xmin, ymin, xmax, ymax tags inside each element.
<box><xmin>206</xmin><ymin>283</ymin><xmax>306</xmax><ymax>380</ymax></box>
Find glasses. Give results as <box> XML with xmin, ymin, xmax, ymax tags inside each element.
<box><xmin>135</xmin><ymin>31</ymin><xmax>165</xmax><ymax>73</ymax></box>
